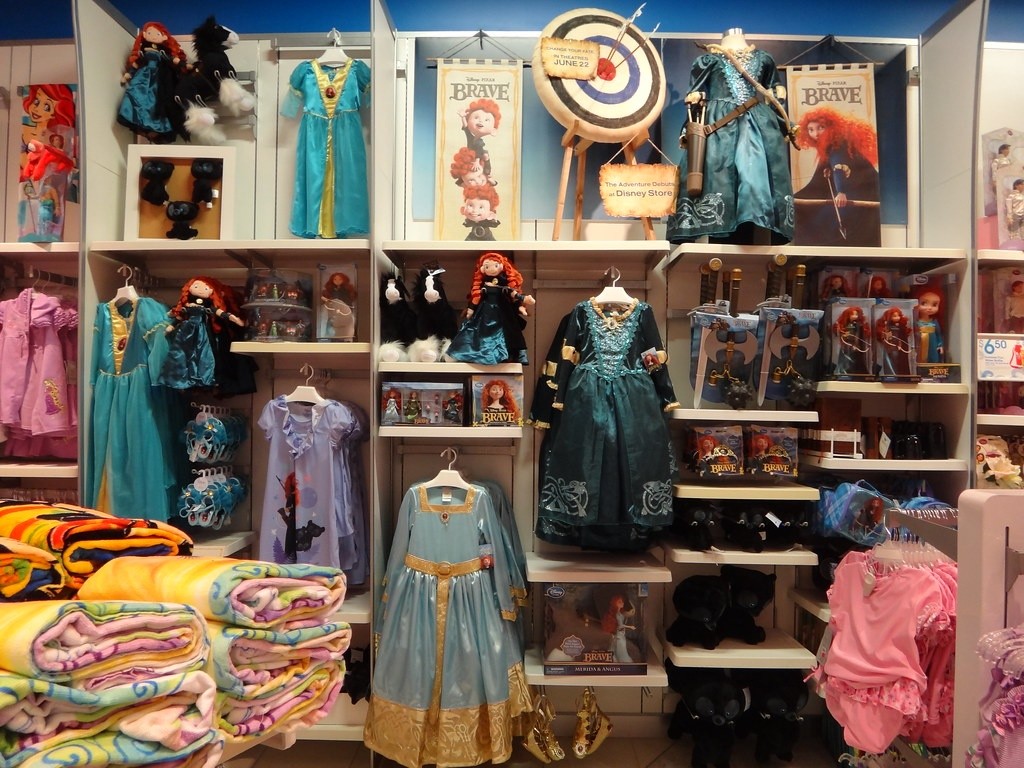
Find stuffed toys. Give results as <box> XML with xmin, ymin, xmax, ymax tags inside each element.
<box><xmin>188</xmin><ymin>20</ymin><xmax>254</xmax><ymax>144</ymax></box>
<box><xmin>384</xmin><ymin>261</ymin><xmax>450</xmax><ymax>362</ymax></box>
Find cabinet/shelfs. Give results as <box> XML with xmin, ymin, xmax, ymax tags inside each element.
<box><xmin>521</xmin><ymin>552</ymin><xmax>669</xmax><ymax>687</ymax></box>
<box><xmin>664</xmin><ymin>247</ymin><xmax>974</xmax><ymax>669</ymax></box>
<box><xmin>376</xmin><ymin>0</ymin><xmax>670</xmax><ymax>439</ymax></box>
<box><xmin>81</xmin><ymin>0</ymin><xmax>375</xmax><ymax>742</ymax></box>
<box><xmin>1</xmin><ymin>1</ymin><xmax>83</xmax><ymax>502</ymax></box>
<box><xmin>975</xmin><ymin>249</ymin><xmax>1024</xmax><ymax>427</ymax></box>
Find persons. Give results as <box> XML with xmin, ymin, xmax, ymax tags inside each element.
<box><xmin>158</xmin><ymin>278</ymin><xmax>245</xmax><ymax>390</ymax></box>
<box><xmin>1002</xmin><ymin>280</ymin><xmax>1024</xmax><ymax>333</ymax></box>
<box><xmin>454</xmin><ymin>253</ymin><xmax>537</xmax><ymax>362</ymax></box>
<box><xmin>321</xmin><ymin>270</ymin><xmax>358</xmax><ymax>341</ymax></box>
<box><xmin>987</xmin><ymin>142</ymin><xmax>1024</xmax><ymax>240</ymax></box>
<box><xmin>823</xmin><ymin>270</ymin><xmax>947</xmax><ymax>382</ymax></box>
<box><xmin>602</xmin><ymin>597</ymin><xmax>637</xmax><ymax>663</ymax></box>
<box><xmin>384</xmin><ymin>390</ymin><xmax>462</xmax><ymax>426</ymax></box>
<box><xmin>115</xmin><ymin>21</ymin><xmax>188</xmax><ymax>142</ymax></box>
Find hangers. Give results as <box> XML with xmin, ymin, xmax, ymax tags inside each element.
<box><xmin>31</xmin><ymin>266</ymin><xmax>74</xmax><ymax>301</ymax></box>
<box><xmin>115</xmin><ymin>265</ymin><xmax>158</xmax><ymax>301</ymax></box>
<box><xmin>286</xmin><ymin>364</ymin><xmax>332</xmax><ymax>405</ymax></box>
<box><xmin>596</xmin><ymin>266</ymin><xmax>633</xmax><ymax>308</ymax></box>
<box><xmin>422</xmin><ymin>446</ymin><xmax>471</xmax><ymax>491</ymax></box>
<box><xmin>868</xmin><ymin>505</ymin><xmax>959</xmax><ymax>579</ymax></box>
<box><xmin>317</xmin><ymin>28</ymin><xmax>350</xmax><ymax>66</ymax></box>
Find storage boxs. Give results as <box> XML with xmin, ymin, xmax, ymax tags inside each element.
<box><xmin>541</xmin><ymin>582</ymin><xmax>648</xmax><ymax>676</ymax></box>
<box><xmin>381</xmin><ymin>380</ymin><xmax>464</xmax><ymax>427</ymax></box>
<box><xmin>468</xmin><ymin>374</ymin><xmax>524</xmax><ymax>427</ymax></box>
